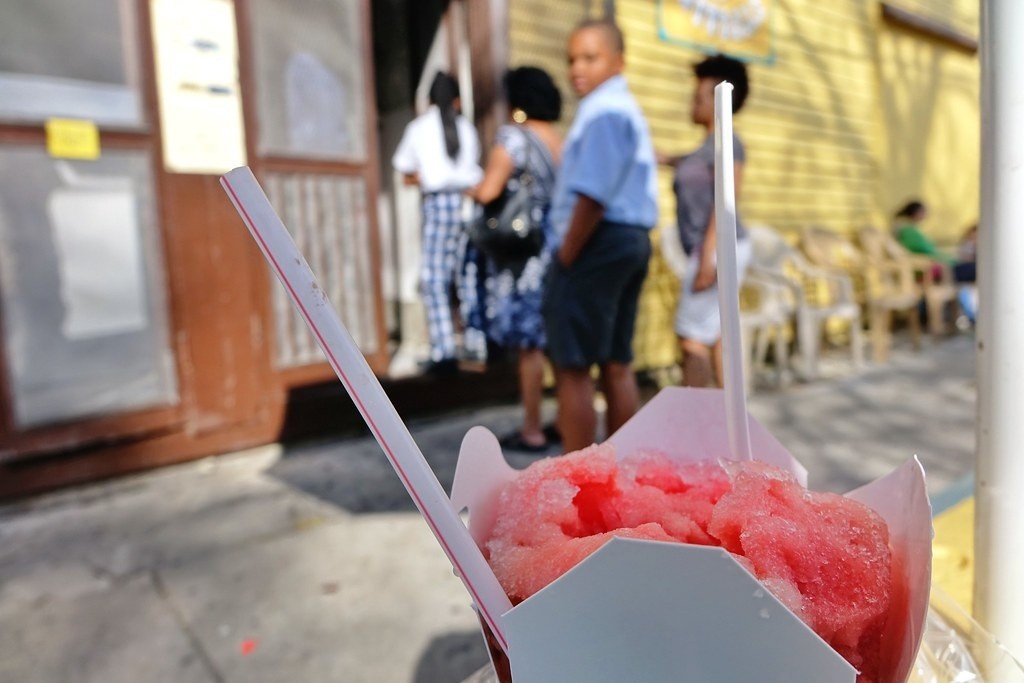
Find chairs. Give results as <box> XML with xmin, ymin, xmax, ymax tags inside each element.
<box><xmin>734</xmin><ymin>207</ymin><xmax>959</xmax><ymax>396</ymax></box>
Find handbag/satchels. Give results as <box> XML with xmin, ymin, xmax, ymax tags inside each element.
<box><xmin>479</xmin><ymin>124</ymin><xmax>544</xmax><ymax>256</ymax></box>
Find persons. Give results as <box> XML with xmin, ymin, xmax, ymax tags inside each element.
<box><xmin>656</xmin><ymin>54</ymin><xmax>750</xmax><ymax>389</ymax></box>
<box><xmin>467</xmin><ymin>65</ymin><xmax>566</xmax><ymax>454</ymax></box>
<box><xmin>390</xmin><ymin>71</ymin><xmax>488</xmax><ymax>375</ymax></box>
<box><xmin>539</xmin><ymin>19</ymin><xmax>659</xmax><ymax>457</ymax></box>
<box><xmin>894</xmin><ymin>202</ymin><xmax>961</xmax><ymax>286</ymax></box>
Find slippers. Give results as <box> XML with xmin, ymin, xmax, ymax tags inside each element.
<box><xmin>543</xmin><ymin>423</ymin><xmax>562</xmax><ymax>443</ymax></box>
<box><xmin>499</xmin><ymin>430</ymin><xmax>547</xmax><ymax>452</ymax></box>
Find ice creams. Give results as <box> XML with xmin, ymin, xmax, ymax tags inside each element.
<box><xmin>483</xmin><ymin>439</ymin><xmax>893</xmax><ymax>683</ymax></box>
